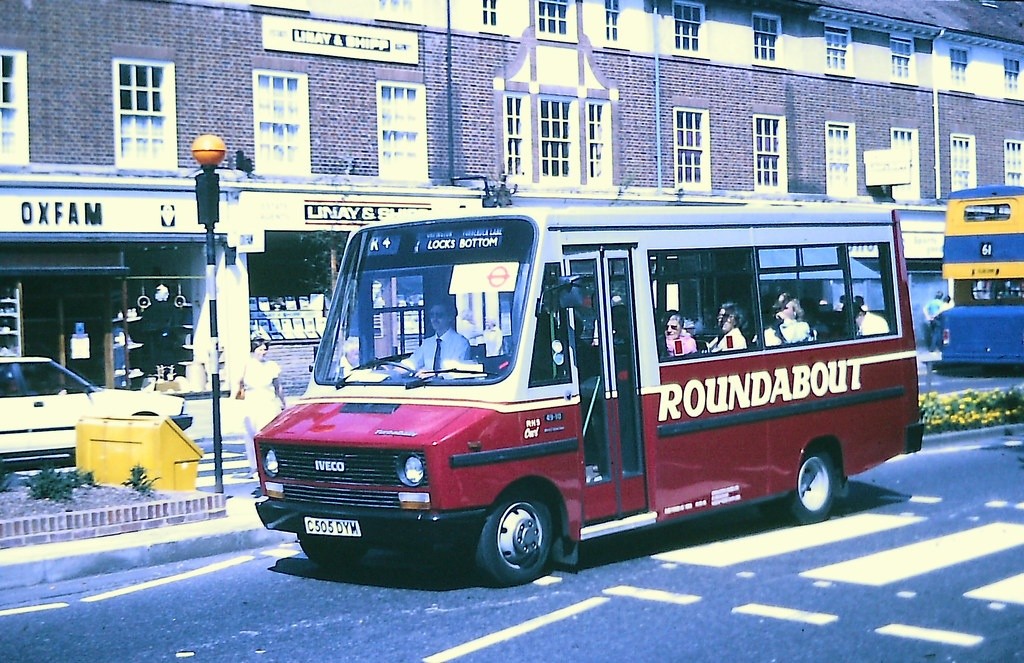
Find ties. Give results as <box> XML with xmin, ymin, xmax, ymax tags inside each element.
<box><xmin>433</xmin><ymin>338</ymin><xmax>443</xmax><ymax>376</ymax></box>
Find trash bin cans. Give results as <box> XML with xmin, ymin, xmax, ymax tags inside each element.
<box><xmin>75</xmin><ymin>415</ymin><xmax>205</xmax><ymax>489</ymax></box>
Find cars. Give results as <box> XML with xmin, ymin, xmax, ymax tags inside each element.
<box><xmin>0</xmin><ymin>356</ymin><xmax>193</xmax><ymax>478</ymax></box>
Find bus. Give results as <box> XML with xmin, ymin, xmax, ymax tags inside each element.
<box><xmin>939</xmin><ymin>184</ymin><xmax>1024</xmax><ymax>372</ymax></box>
<box><xmin>252</xmin><ymin>202</ymin><xmax>931</xmax><ymax>590</ymax></box>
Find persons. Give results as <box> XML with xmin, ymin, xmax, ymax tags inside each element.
<box><xmin>228</xmin><ymin>330</ymin><xmax>289</xmax><ymax>484</ymax></box>
<box><xmin>392</xmin><ymin>292</ymin><xmax>953</xmax><ymax>382</ymax></box>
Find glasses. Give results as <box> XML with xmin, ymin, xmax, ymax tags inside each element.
<box><xmin>430</xmin><ymin>312</ymin><xmax>447</xmax><ymax>319</ymax></box>
<box><xmin>664</xmin><ymin>324</ymin><xmax>678</xmax><ymax>331</ymax></box>
